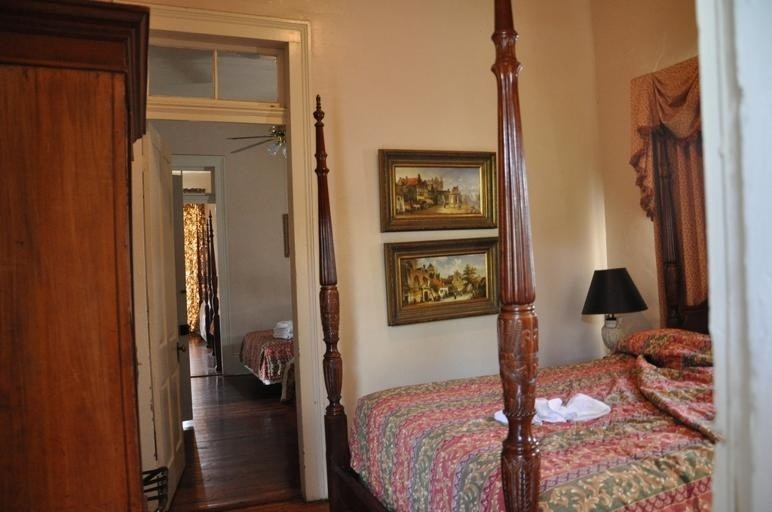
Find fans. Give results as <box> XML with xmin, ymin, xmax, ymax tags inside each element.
<box><xmin>225</xmin><ymin>124</ymin><xmax>287</xmax><ymax>156</ymax></box>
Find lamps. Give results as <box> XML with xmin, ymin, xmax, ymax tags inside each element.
<box><xmin>266</xmin><ymin>140</ymin><xmax>286</xmax><ymax>157</ymax></box>
<box><xmin>580</xmin><ymin>267</ymin><xmax>647</xmax><ymax>351</ymax></box>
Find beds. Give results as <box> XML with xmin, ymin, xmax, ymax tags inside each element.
<box><xmin>240</xmin><ymin>329</ymin><xmax>295</xmax><ymax>402</ymax></box>
<box><xmin>349</xmin><ymin>328</ymin><xmax>713</xmax><ymax>511</ymax></box>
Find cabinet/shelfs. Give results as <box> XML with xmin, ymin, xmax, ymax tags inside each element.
<box><xmin>0</xmin><ymin>2</ymin><xmax>152</xmax><ymax>512</ymax></box>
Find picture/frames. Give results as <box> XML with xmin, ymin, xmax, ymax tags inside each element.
<box><xmin>378</xmin><ymin>147</ymin><xmax>501</xmax><ymax>328</ymax></box>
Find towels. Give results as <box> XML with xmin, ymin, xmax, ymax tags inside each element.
<box><xmin>273</xmin><ymin>320</ymin><xmax>294</xmax><ymax>340</ymax></box>
<box><xmin>493</xmin><ymin>389</ymin><xmax>613</xmax><ymax>428</ymax></box>
